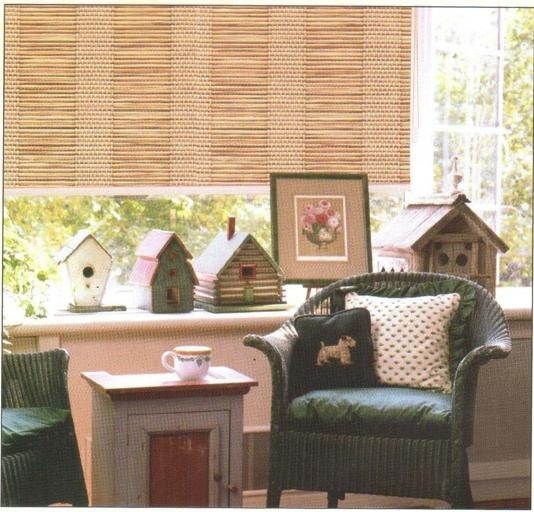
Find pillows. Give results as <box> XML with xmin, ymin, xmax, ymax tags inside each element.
<box><xmin>294</xmin><ymin>307</ymin><xmax>380</xmax><ymax>392</ymax></box>
<box><xmin>344</xmin><ymin>290</ymin><xmax>462</xmax><ymax>394</ymax></box>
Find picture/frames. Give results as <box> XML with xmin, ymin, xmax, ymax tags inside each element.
<box><xmin>269</xmin><ymin>171</ymin><xmax>373</xmax><ymax>285</ymax></box>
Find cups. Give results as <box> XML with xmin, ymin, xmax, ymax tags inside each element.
<box><xmin>161</xmin><ymin>346</ymin><xmax>212</xmax><ymax>381</ymax></box>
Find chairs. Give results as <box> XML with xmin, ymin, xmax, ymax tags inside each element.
<box><xmin>243</xmin><ymin>271</ymin><xmax>511</xmax><ymax>509</ymax></box>
<box><xmin>1</xmin><ymin>348</ymin><xmax>89</xmax><ymax>507</ymax></box>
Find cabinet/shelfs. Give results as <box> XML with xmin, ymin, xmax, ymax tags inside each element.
<box><xmin>79</xmin><ymin>365</ymin><xmax>258</xmax><ymax>507</ymax></box>
